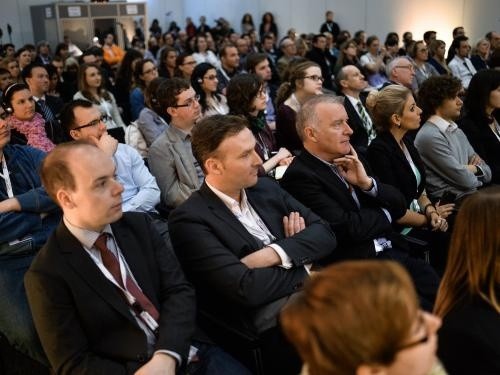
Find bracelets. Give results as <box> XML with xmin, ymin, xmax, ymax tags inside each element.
<box><xmin>428</xmin><ymin>210</ymin><xmax>439</xmax><ymax>220</ymax></box>
<box><xmin>424</xmin><ymin>203</ymin><xmax>434</xmax><ymax>215</ymax></box>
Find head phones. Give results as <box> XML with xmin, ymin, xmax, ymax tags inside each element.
<box><xmin>3</xmin><ymin>82</ymin><xmax>18</xmax><ymax>112</ymax></box>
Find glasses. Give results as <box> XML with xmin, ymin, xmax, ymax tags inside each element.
<box><xmin>398</xmin><ymin>314</ymin><xmax>431</xmax><ymax>352</ymax></box>
<box><xmin>444</xmin><ymin>92</ymin><xmax>463</xmax><ymax>99</ymax></box>
<box><xmin>72</xmin><ymin>115</ymin><xmax>107</xmax><ymax>130</ymax></box>
<box><xmin>179</xmin><ymin>61</ymin><xmax>197</xmax><ymax>66</ymax></box>
<box><xmin>283</xmin><ymin>42</ymin><xmax>296</xmax><ymax>48</ymax></box>
<box><xmin>460</xmin><ymin>45</ymin><xmax>470</xmax><ymax>49</ymax></box>
<box><xmin>169</xmin><ymin>94</ymin><xmax>200</xmax><ymax>107</ymax></box>
<box><xmin>349</xmin><ymin>46</ymin><xmax>358</xmax><ymax>50</ymax></box>
<box><xmin>394</xmin><ymin>65</ymin><xmax>416</xmax><ymax>70</ymax></box>
<box><xmin>417</xmin><ymin>47</ymin><xmax>428</xmax><ymax>53</ymax></box>
<box><xmin>303</xmin><ymin>75</ymin><xmax>324</xmax><ymax>81</ymax></box>
<box><xmin>254</xmin><ymin>87</ymin><xmax>266</xmax><ymax>97</ymax></box>
<box><xmin>203</xmin><ymin>75</ymin><xmax>219</xmax><ymax>80</ymax></box>
<box><xmin>0</xmin><ymin>112</ymin><xmax>9</xmax><ymax>120</ymax></box>
<box><xmin>143</xmin><ymin>66</ymin><xmax>158</xmax><ymax>75</ymax></box>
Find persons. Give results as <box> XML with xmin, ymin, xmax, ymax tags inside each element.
<box><xmin>279</xmin><ymin>259</ymin><xmax>443</xmax><ymax>375</ymax></box>
<box><xmin>24</xmin><ymin>138</ymin><xmax>250</xmax><ymax>375</ymax></box>
<box><xmin>0</xmin><ymin>11</ymin><xmax>500</xmax><ymax>256</ymax></box>
<box><xmin>413</xmin><ymin>74</ymin><xmax>492</xmax><ymax>199</ymax></box>
<box><xmin>0</xmin><ymin>106</ymin><xmax>66</xmax><ymax>368</ymax></box>
<box><xmin>434</xmin><ymin>186</ymin><xmax>500</xmax><ymax>375</ymax></box>
<box><xmin>365</xmin><ymin>83</ymin><xmax>457</xmax><ymax>268</ymax></box>
<box><xmin>281</xmin><ymin>96</ymin><xmax>442</xmax><ymax>311</ymax></box>
<box><xmin>455</xmin><ymin>70</ymin><xmax>499</xmax><ymax>185</ymax></box>
<box><xmin>169</xmin><ymin>113</ymin><xmax>337</xmax><ymax>375</ymax></box>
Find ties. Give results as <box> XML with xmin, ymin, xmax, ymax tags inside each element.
<box><xmin>331</xmin><ymin>165</ymin><xmax>389</xmax><ymax>248</ymax></box>
<box><xmin>39</xmin><ymin>100</ymin><xmax>53</xmax><ymax>121</ymax></box>
<box><xmin>91</xmin><ymin>232</ymin><xmax>160</xmax><ymax>338</ymax></box>
<box><xmin>463</xmin><ymin>62</ymin><xmax>474</xmax><ymax>77</ymax></box>
<box><xmin>0</xmin><ymin>161</ymin><xmax>9</xmax><ymax>202</ymax></box>
<box><xmin>357</xmin><ymin>102</ymin><xmax>376</xmax><ymax>140</ymax></box>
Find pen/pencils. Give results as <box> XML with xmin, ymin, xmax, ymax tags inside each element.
<box><xmin>271</xmin><ymin>151</ymin><xmax>278</xmax><ymax>154</ymax></box>
